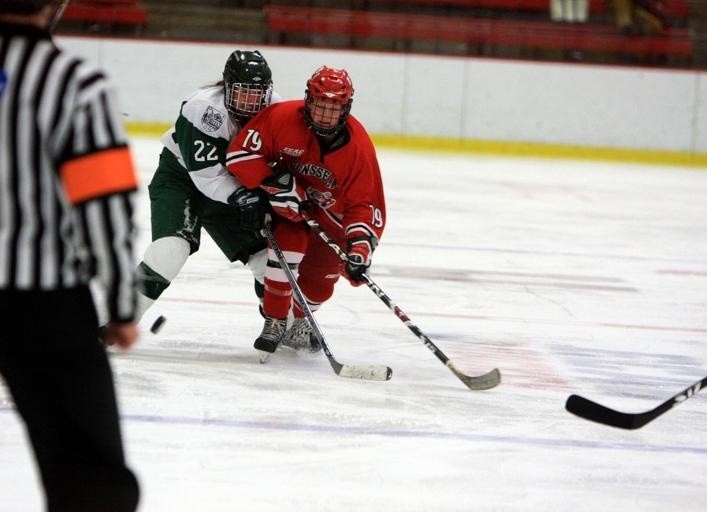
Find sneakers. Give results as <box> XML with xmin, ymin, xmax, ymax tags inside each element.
<box><xmin>98</xmin><ymin>319</ymin><xmax>116</xmax><ymax>349</ymax></box>
<box><xmin>254</xmin><ymin>316</ymin><xmax>288</xmax><ymax>353</ymax></box>
<box><xmin>282</xmin><ymin>317</ymin><xmax>323</xmax><ymax>352</ymax></box>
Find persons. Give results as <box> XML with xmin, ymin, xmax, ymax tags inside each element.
<box><xmin>602</xmin><ymin>0</ymin><xmax>673</xmax><ymax>69</ymax></box>
<box><xmin>1</xmin><ymin>1</ymin><xmax>142</xmax><ymax>512</ymax></box>
<box><xmin>225</xmin><ymin>65</ymin><xmax>388</xmax><ymax>356</ymax></box>
<box><xmin>98</xmin><ymin>46</ymin><xmax>293</xmax><ymax>349</ymax></box>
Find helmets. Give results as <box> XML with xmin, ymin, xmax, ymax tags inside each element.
<box><xmin>303</xmin><ymin>65</ymin><xmax>355</xmax><ymax>139</ymax></box>
<box><xmin>222</xmin><ymin>49</ymin><xmax>273</xmax><ymax>124</ymax></box>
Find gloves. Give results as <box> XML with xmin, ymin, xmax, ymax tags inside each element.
<box><xmin>257</xmin><ymin>170</ymin><xmax>314</xmax><ymax>222</ymax></box>
<box><xmin>227</xmin><ymin>186</ymin><xmax>275</xmax><ymax>237</ymax></box>
<box><xmin>338</xmin><ymin>236</ymin><xmax>378</xmax><ymax>288</ymax></box>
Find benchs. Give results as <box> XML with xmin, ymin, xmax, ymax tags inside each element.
<box><xmin>62</xmin><ymin>5</ymin><xmax>151</xmax><ymax>36</ymax></box>
<box><xmin>266</xmin><ymin>1</ymin><xmax>695</xmax><ymax>56</ymax></box>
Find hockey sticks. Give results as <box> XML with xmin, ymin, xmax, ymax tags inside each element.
<box><xmin>264</xmin><ymin>227</ymin><xmax>393</xmax><ymax>382</ymax></box>
<box><xmin>563</xmin><ymin>378</ymin><xmax>707</xmax><ymax>430</ymax></box>
<box><xmin>298</xmin><ymin>206</ymin><xmax>500</xmax><ymax>391</ymax></box>
